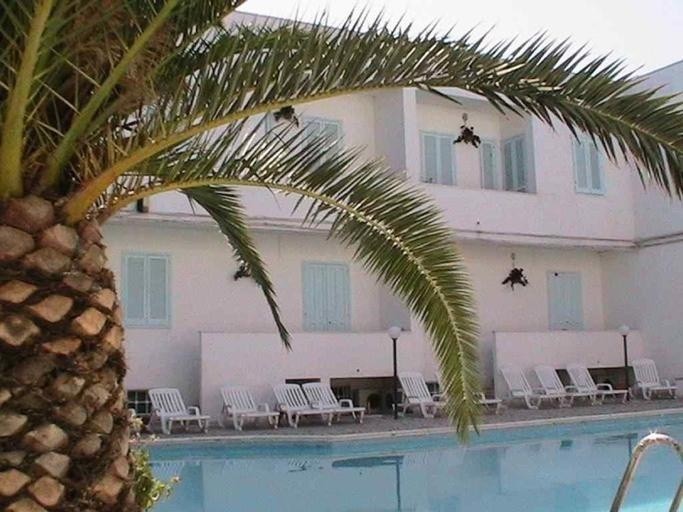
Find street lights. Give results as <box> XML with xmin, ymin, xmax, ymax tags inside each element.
<box><xmin>384</xmin><ymin>325</ymin><xmax>403</xmax><ymax>421</ymax></box>
<box><xmin>616</xmin><ymin>323</ymin><xmax>634</xmax><ymax>401</ymax></box>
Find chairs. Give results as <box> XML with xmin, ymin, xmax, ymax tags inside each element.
<box><xmin>396</xmin><ymin>358</ymin><xmax>679</xmax><ymax>419</ymax></box>
<box><xmin>146</xmin><ymin>381</ymin><xmax>366</xmax><ymax>435</ymax></box>
